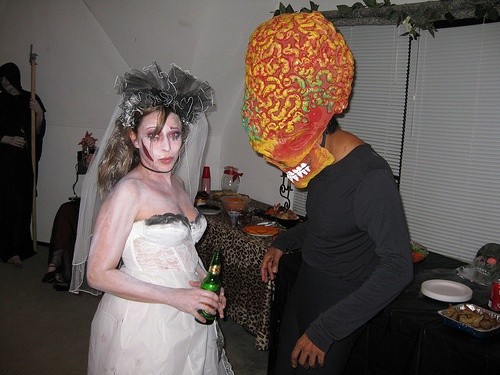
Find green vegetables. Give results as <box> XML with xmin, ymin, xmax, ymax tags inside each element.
<box><xmin>410</xmin><ymin>243</ymin><xmax>427</xmax><ymax>254</ymax></box>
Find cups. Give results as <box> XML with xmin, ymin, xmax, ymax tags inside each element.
<box><xmin>82</xmin><ymin>142</ymin><xmax>95</xmax><ymax>156</ymax></box>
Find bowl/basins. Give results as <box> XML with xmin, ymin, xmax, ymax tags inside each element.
<box><xmin>410</xmin><ymin>241</ymin><xmax>429</xmax><ymax>263</ymax></box>
<box><xmin>438</xmin><ymin>303</ymin><xmax>500</xmax><ymax>339</ymax></box>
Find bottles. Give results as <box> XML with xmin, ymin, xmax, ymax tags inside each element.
<box><xmin>195</xmin><ymin>191</ymin><xmax>208</xmax><ymax>205</ymax></box>
<box><xmin>202</xmin><ymin>166</ymin><xmax>210</xmax><ymax>195</ymax></box>
<box><xmin>195</xmin><ymin>249</ymin><xmax>222</xmax><ymax>325</ymax></box>
<box><xmin>18</xmin><ymin>128</ymin><xmax>29</xmax><ymax>150</ymax></box>
<box><xmin>221</xmin><ymin>166</ymin><xmax>240</xmax><ymax>194</ymax></box>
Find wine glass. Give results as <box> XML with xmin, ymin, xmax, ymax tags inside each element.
<box><xmin>220</xmin><ymin>195</ymin><xmax>250</xmax><ymax>230</ymax></box>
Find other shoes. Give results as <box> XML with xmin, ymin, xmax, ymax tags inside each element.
<box><xmin>41</xmin><ymin>265</ymin><xmax>60</xmax><ymax>283</ymax></box>
<box><xmin>54</xmin><ymin>278</ymin><xmax>70</xmax><ymax>291</ymax></box>
<box><xmin>7</xmin><ymin>256</ymin><xmax>24</xmax><ymax>267</ymax></box>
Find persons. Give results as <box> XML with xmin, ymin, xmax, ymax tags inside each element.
<box><xmin>69</xmin><ymin>58</ymin><xmax>235</xmax><ymax>375</ymax></box>
<box><xmin>0</xmin><ymin>62</ymin><xmax>100</xmax><ymax>290</ymax></box>
<box><xmin>244</xmin><ymin>4</ymin><xmax>416</xmax><ymax>375</ymax></box>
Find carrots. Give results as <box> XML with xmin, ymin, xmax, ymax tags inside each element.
<box><xmin>411</xmin><ymin>252</ymin><xmax>425</xmax><ymax>262</ymax></box>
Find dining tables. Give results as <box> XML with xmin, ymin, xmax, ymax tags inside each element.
<box><xmin>265</xmin><ymin>251</ymin><xmax>500</xmax><ymax>375</ymax></box>
<box><xmin>195</xmin><ymin>190</ymin><xmax>294</xmax><ymax>351</ymax></box>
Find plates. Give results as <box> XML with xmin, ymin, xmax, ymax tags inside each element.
<box><xmin>254</xmin><ymin>205</ymin><xmax>304</xmax><ymax>228</ymax></box>
<box><xmin>243</xmin><ymin>225</ymin><xmax>279</xmax><ymax>237</ymax></box>
<box><xmin>420</xmin><ymin>279</ymin><xmax>472</xmax><ymax>302</ymax></box>
<box><xmin>197</xmin><ymin>205</ymin><xmax>222</xmax><ymax>215</ymax></box>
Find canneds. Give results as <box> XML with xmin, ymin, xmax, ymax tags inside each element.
<box><xmin>488</xmin><ymin>279</ymin><xmax>500</xmax><ymax>312</ymax></box>
